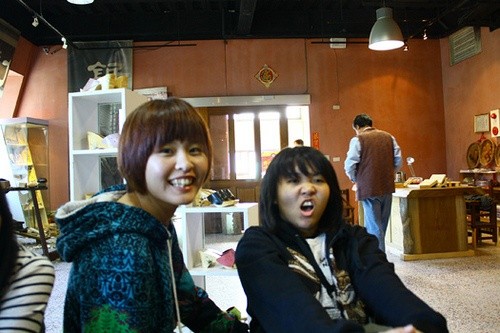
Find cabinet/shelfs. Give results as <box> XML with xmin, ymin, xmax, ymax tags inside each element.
<box><xmin>68</xmin><ymin>87</ymin><xmax>258</xmax><ymax>277</ymax></box>
<box><xmin>0</xmin><ymin>117</ymin><xmax>50</xmax><ymax>232</ymax></box>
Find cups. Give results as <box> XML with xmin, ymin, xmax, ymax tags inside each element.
<box><xmin>207</xmin><ymin>188</ymin><xmax>236</xmax><ymax>205</ymax></box>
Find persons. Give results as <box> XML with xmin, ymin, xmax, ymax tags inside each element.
<box><xmin>344</xmin><ymin>114</ymin><xmax>402</xmax><ymax>252</ymax></box>
<box><xmin>292</xmin><ymin>139</ymin><xmax>304</xmax><ymax>148</ymax></box>
<box><xmin>473</xmin><ymin>149</ymin><xmax>478</xmax><ymax>165</ymax></box>
<box><xmin>54</xmin><ymin>98</ymin><xmax>250</xmax><ymax>333</ymax></box>
<box><xmin>0</xmin><ymin>187</ymin><xmax>55</xmax><ymax>333</ymax></box>
<box><xmin>235</xmin><ymin>147</ymin><xmax>448</xmax><ymax>333</ymax></box>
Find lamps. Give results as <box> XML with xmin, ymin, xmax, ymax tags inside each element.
<box><xmin>402</xmin><ymin>22</ymin><xmax>429</xmax><ymax>51</ymax></box>
<box><xmin>62</xmin><ymin>37</ymin><xmax>67</xmax><ymax>49</ymax></box>
<box><xmin>368</xmin><ymin>0</ymin><xmax>404</xmax><ymax>51</ymax></box>
<box><xmin>31</xmin><ymin>17</ymin><xmax>39</xmax><ymax>27</ymax></box>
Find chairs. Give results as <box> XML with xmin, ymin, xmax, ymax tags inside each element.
<box><xmin>341</xmin><ymin>189</ymin><xmax>354</xmax><ymax>223</ymax></box>
<box><xmin>465</xmin><ymin>173</ymin><xmax>497</xmax><ymax>244</ymax></box>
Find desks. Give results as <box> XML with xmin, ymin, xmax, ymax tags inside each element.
<box><xmin>351</xmin><ymin>185</ymin><xmax>480</xmax><ymax>260</ymax></box>
